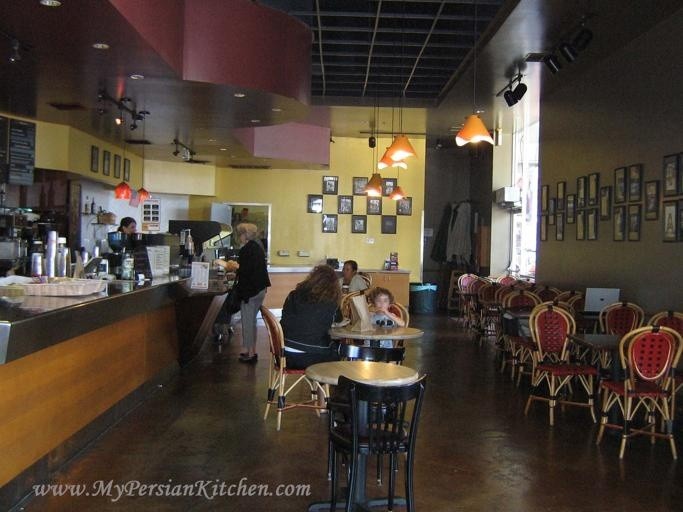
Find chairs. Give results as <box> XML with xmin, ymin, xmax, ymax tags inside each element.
<box><xmin>256</xmin><ymin>296</ymin><xmax>426</xmax><ymax>512</ymax></box>
<box><xmin>455</xmin><ymin>273</ymin><xmax>683</xmax><ymax>463</ymax></box>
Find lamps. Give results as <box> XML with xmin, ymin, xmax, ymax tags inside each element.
<box><xmin>360</xmin><ymin>56</ymin><xmax>383</xmax><ymax>199</ymax></box>
<box><xmin>544</xmin><ymin>44</ymin><xmax>559</xmax><ymax>73</ymax></box>
<box><xmin>136</xmin><ymin>109</ymin><xmax>151</xmax><ymax>200</ymax></box>
<box><xmin>560</xmin><ymin>11</ymin><xmax>597</xmax><ymax>64</ymax></box>
<box><xmin>391</xmin><ymin>1</ymin><xmax>418</xmax><ymax>160</ymax></box>
<box><xmin>115</xmin><ymin>96</ymin><xmax>132</xmax><ymax>201</ymax></box>
<box><xmin>169</xmin><ymin>136</ymin><xmax>194</xmax><ymax>160</ymax></box>
<box><xmin>502</xmin><ymin>77</ymin><xmax>525</xmax><ymax>108</ymax></box>
<box><xmin>380</xmin><ymin>2</ymin><xmax>406</xmax><ymax>169</ymax></box>
<box><xmin>386</xmin><ymin>31</ymin><xmax>407</xmax><ymax>203</ymax></box>
<box><xmin>6</xmin><ymin>36</ymin><xmax>21</xmax><ymax>65</ymax></box>
<box><xmin>454</xmin><ymin>0</ymin><xmax>494</xmax><ymax>150</ymax></box>
<box><xmin>95</xmin><ymin>89</ymin><xmax>144</xmax><ymax>131</ymax></box>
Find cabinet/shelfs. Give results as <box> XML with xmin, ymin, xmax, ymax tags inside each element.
<box><xmin>81</xmin><ymin>208</ymin><xmax>119</xmax><ymax>237</ymax></box>
<box><xmin>472</xmin><ymin>223</ymin><xmax>490</xmax><ymax>268</ymax></box>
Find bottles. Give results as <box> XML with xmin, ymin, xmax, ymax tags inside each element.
<box><xmin>48</xmin><ymin>180</ymin><xmax>56</xmax><ymax>208</ymax></box>
<box><xmin>184</xmin><ymin>229</ymin><xmax>195</xmax><ymax>265</ymax></box>
<box><xmin>229</xmin><ymin>246</ymin><xmax>235</xmax><ymax>256</ymax></box>
<box><xmin>0</xmin><ymin>182</ymin><xmax>9</xmax><ymax>208</ymax></box>
<box><xmin>96</xmin><ymin>205</ymin><xmax>103</xmax><ymax>223</ymax></box>
<box><xmin>84</xmin><ymin>195</ymin><xmax>91</xmax><ymax>213</ymax></box>
<box><xmin>39</xmin><ymin>184</ymin><xmax>46</xmax><ymax>207</ymax></box>
<box><xmin>90</xmin><ymin>196</ymin><xmax>97</xmax><ymax>213</ymax></box>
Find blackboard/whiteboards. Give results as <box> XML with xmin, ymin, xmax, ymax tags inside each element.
<box><xmin>0</xmin><ymin>115</ymin><xmax>36</xmax><ymax>188</ymax></box>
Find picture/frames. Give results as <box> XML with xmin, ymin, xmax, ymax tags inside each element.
<box><xmin>113</xmin><ymin>153</ymin><xmax>121</xmax><ymax>179</ymax></box>
<box><xmin>301</xmin><ymin>174</ymin><xmax>413</xmax><ymax>236</ymax></box>
<box><xmin>89</xmin><ymin>145</ymin><xmax>98</xmax><ymax>174</ymax></box>
<box><xmin>101</xmin><ymin>149</ymin><xmax>111</xmax><ymax>176</ymax></box>
<box><xmin>123</xmin><ymin>158</ymin><xmax>130</xmax><ymax>181</ymax></box>
<box><xmin>539</xmin><ymin>151</ymin><xmax>682</xmax><ymax>247</ymax></box>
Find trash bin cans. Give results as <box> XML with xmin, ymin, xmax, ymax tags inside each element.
<box><xmin>409</xmin><ymin>282</ymin><xmax>439</xmax><ymax>315</ymax></box>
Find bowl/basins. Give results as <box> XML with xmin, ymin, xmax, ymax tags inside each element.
<box><xmin>101</xmin><ymin>212</ymin><xmax>115</xmax><ymax>224</ymax></box>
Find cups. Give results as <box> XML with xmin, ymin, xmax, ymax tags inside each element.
<box><xmin>342</xmin><ymin>286</ymin><xmax>349</xmax><ymax>295</ymax></box>
<box><xmin>169</xmin><ymin>264</ymin><xmax>180</xmax><ymax>280</ymax></box>
<box><xmin>29</xmin><ymin>231</ymin><xmax>101</xmax><ymax>280</ymax></box>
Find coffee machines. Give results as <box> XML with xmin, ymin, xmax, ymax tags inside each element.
<box><xmin>105</xmin><ymin>231</ymin><xmax>131</xmax><ymax>280</ymax></box>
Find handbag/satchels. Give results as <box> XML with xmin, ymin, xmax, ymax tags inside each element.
<box><xmin>349</xmin><ymin>291</ymin><xmax>374</xmax><ymax>332</ymax></box>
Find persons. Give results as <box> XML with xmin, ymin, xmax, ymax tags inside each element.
<box><xmin>226</xmin><ymin>223</ymin><xmax>272</xmax><ymax>363</ymax></box>
<box><xmin>278</xmin><ymin>265</ymin><xmax>343</xmax><ymax>413</ymax></box>
<box><xmin>116</xmin><ymin>217</ymin><xmax>137</xmax><ymax>233</ymax></box>
<box><xmin>338</xmin><ymin>261</ymin><xmax>367</xmax><ymax>294</ymax></box>
<box><xmin>362</xmin><ymin>289</ymin><xmax>404</xmax><ymax>365</ymax></box>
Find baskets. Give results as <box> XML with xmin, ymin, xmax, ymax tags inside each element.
<box><xmin>16</xmin><ymin>277</ymin><xmax>107</xmax><ymax>296</ymax></box>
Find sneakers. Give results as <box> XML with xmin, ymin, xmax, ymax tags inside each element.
<box><xmin>239</xmin><ymin>351</ymin><xmax>257</xmax><ymax>356</ymax></box>
<box><xmin>237</xmin><ymin>354</ymin><xmax>258</xmax><ymax>366</ymax></box>
